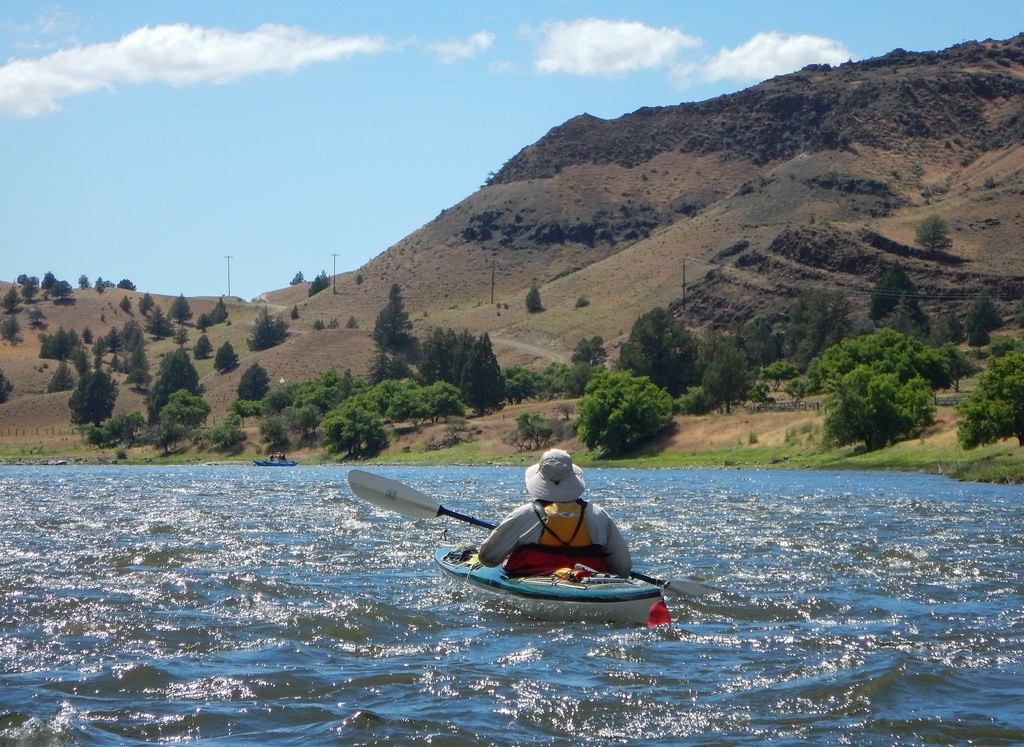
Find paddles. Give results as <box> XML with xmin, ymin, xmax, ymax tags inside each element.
<box><xmin>345</xmin><ymin>463</ymin><xmax>721</xmax><ymax>597</ymax></box>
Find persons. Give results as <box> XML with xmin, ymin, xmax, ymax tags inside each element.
<box><xmin>270</xmin><ymin>452</ymin><xmax>286</xmax><ymax>462</ymax></box>
<box><xmin>478</xmin><ymin>449</ymin><xmax>633</xmax><ymax>578</ymax></box>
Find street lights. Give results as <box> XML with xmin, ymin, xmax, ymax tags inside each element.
<box><xmin>225</xmin><ymin>256</ymin><xmax>232</xmax><ymax>296</ymax></box>
<box><xmin>330</xmin><ymin>253</ymin><xmax>340</xmax><ymax>293</ymax></box>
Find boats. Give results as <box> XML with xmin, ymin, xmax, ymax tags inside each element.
<box><xmin>432</xmin><ymin>541</ymin><xmax>664</xmax><ymax>625</ymax></box>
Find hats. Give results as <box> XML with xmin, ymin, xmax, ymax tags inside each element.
<box><xmin>525</xmin><ymin>448</ymin><xmax>586</xmax><ymax>502</ymax></box>
